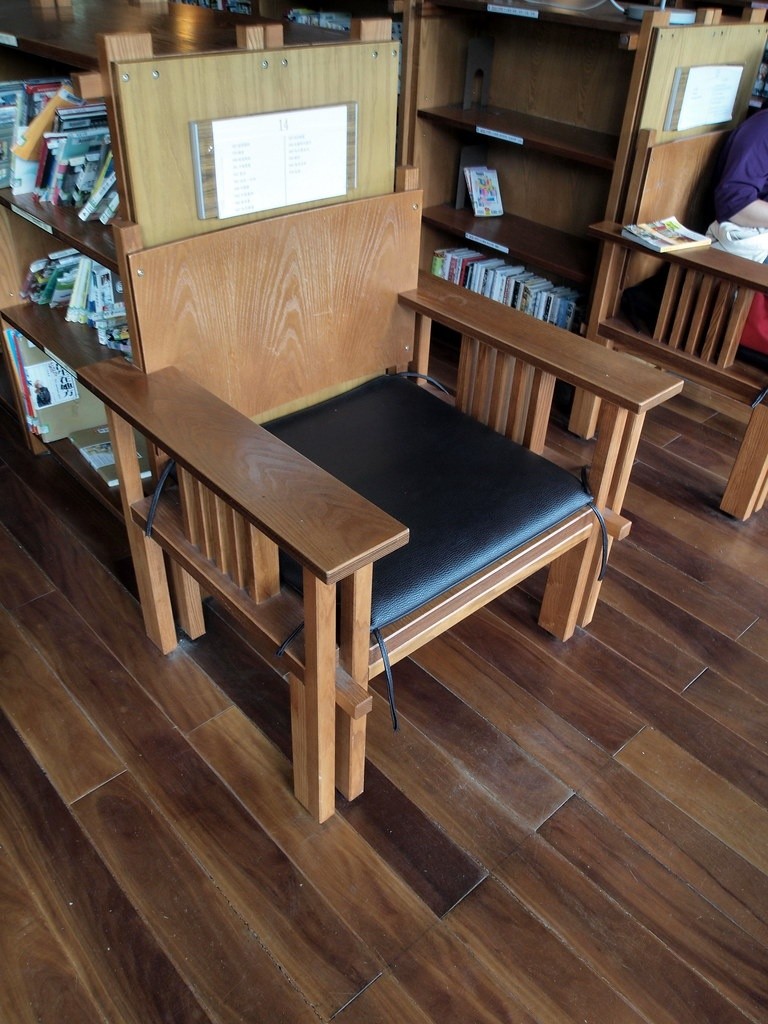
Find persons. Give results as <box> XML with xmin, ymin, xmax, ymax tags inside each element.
<box><xmin>711</xmin><ymin>108</ymin><xmax>768</xmax><ymax>354</ymax></box>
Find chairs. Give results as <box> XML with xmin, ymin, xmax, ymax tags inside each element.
<box><xmin>75</xmin><ymin>164</ymin><xmax>684</xmax><ymax>825</ymax></box>
<box><xmin>566</xmin><ymin>126</ymin><xmax>768</xmax><ymax>522</ymax></box>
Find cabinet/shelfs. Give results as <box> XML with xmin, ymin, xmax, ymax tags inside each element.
<box><xmin>0</xmin><ymin>0</ymin><xmax>400</xmax><ymax>520</ymax></box>
<box><xmin>409</xmin><ymin>0</ymin><xmax>768</xmax><ymax>350</ymax></box>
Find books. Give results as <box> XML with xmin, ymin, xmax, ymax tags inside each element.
<box><xmin>622</xmin><ymin>216</ymin><xmax>711</xmax><ymax>252</ymax></box>
<box><xmin>0</xmin><ymin>78</ymin><xmax>152</xmax><ymax>486</ymax></box>
<box><xmin>752</xmin><ymin>44</ymin><xmax>768</xmax><ymax>97</ymax></box>
<box><xmin>432</xmin><ymin>247</ymin><xmax>588</xmax><ymax>337</ymax></box>
<box><xmin>184</xmin><ymin>0</ymin><xmax>400</xmax><ymax>93</ymax></box>
<box><xmin>463</xmin><ymin>166</ymin><xmax>503</xmax><ymax>217</ymax></box>
